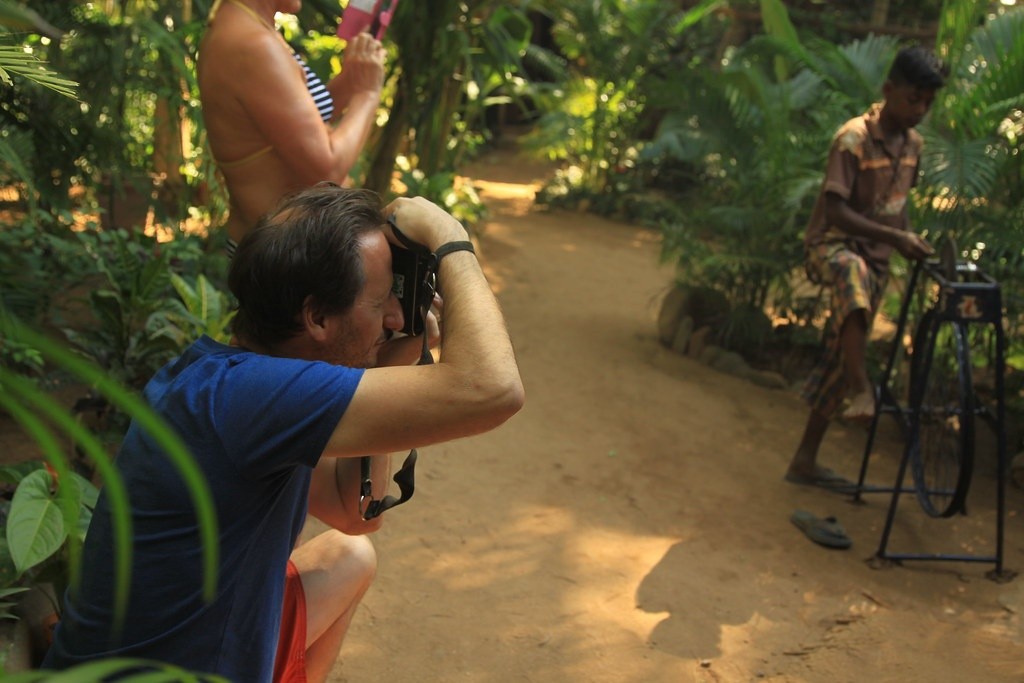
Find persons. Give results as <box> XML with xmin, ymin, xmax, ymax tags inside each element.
<box><xmin>40</xmin><ymin>182</ymin><xmax>527</xmax><ymax>683</ymax></box>
<box><xmin>197</xmin><ymin>0</ymin><xmax>389</xmax><ymax>268</ymax></box>
<box><xmin>784</xmin><ymin>44</ymin><xmax>948</xmax><ymax>491</ymax></box>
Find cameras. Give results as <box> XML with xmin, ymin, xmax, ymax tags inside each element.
<box><xmin>387</xmin><ymin>243</ymin><xmax>437</xmax><ymax>337</ymax></box>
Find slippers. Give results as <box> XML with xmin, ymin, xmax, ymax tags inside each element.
<box><xmin>784</xmin><ymin>467</ymin><xmax>857</xmax><ymax>494</ymax></box>
<box><xmin>791</xmin><ymin>510</ymin><xmax>853</xmax><ymax>547</ymax></box>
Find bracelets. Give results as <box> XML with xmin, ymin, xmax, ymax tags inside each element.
<box><xmin>424</xmin><ymin>240</ymin><xmax>475</xmax><ymax>283</ymax></box>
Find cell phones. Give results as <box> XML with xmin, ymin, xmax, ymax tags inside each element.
<box><xmin>368</xmin><ymin>0</ymin><xmax>398</xmax><ymax>41</ymax></box>
